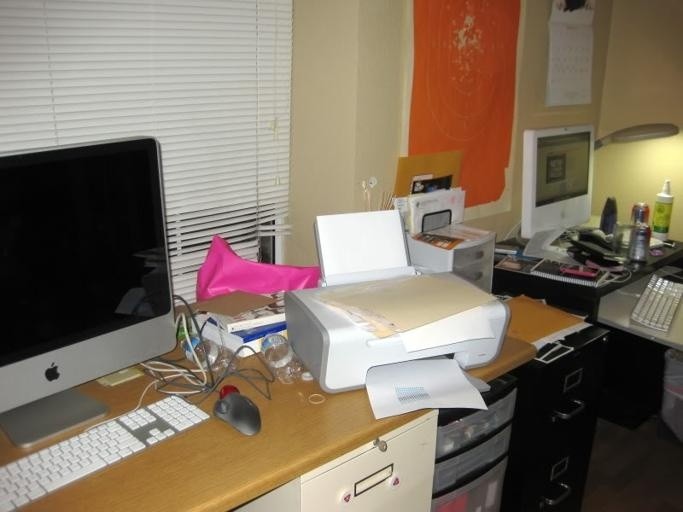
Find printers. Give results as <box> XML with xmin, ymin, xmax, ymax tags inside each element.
<box><xmin>282</xmin><ymin>210</ymin><xmax>511</xmax><ymax>395</ymax></box>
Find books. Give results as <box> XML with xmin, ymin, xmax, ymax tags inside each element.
<box><xmin>190</xmin><ymin>318</ymin><xmax>289</xmax><ymax>358</ymax></box>
<box><xmin>207</xmin><ymin>290</ymin><xmax>286</xmax><ymax>333</ymax></box>
<box><xmin>207</xmin><ymin>318</ymin><xmax>289</xmax><ymax>343</ymax></box>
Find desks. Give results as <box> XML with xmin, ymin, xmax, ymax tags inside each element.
<box><xmin>0</xmin><ymin>288</ymin><xmax>537</xmax><ymax>512</ymax></box>
<box><xmin>499</xmin><ymin>226</ymin><xmax>683</xmax><ymax>428</ymax></box>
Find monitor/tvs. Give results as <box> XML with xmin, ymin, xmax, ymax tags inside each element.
<box><xmin>0</xmin><ymin>134</ymin><xmax>177</xmax><ymax>448</ymax></box>
<box><xmin>522</xmin><ymin>125</ymin><xmax>595</xmax><ymax>239</ymax></box>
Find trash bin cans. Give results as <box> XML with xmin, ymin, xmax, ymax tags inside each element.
<box><xmin>661</xmin><ymin>348</ymin><xmax>683</xmax><ymax>443</ymax></box>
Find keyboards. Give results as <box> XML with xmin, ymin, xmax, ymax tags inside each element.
<box><xmin>0</xmin><ymin>394</ymin><xmax>212</xmax><ymax>511</ymax></box>
<box><xmin>630</xmin><ymin>274</ymin><xmax>683</xmax><ymax>332</ymax></box>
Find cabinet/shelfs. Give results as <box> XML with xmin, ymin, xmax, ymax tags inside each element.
<box><xmin>506</xmin><ymin>293</ymin><xmax>608</xmax><ymax>512</ymax></box>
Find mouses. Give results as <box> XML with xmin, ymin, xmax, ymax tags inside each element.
<box><xmin>213</xmin><ymin>393</ymin><xmax>261</xmax><ymax>436</ymax></box>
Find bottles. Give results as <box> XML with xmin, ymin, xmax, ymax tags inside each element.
<box><xmin>260</xmin><ymin>333</ymin><xmax>304</xmax><ymax>384</ymax></box>
<box><xmin>630</xmin><ymin>202</ymin><xmax>649</xmax><ymax>228</ymax></box>
<box><xmin>651</xmin><ymin>180</ymin><xmax>674</xmax><ymax>242</ymax></box>
<box><xmin>179</xmin><ymin>335</ymin><xmax>239</xmax><ymax>376</ymax></box>
<box><xmin>628</xmin><ymin>224</ymin><xmax>651</xmax><ymax>262</ymax></box>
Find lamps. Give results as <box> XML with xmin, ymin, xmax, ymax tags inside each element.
<box><xmin>594</xmin><ymin>122</ymin><xmax>679</xmax><ymax>155</ymax></box>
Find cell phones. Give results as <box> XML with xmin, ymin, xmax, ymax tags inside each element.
<box><xmin>560</xmin><ymin>262</ymin><xmax>600</xmax><ymax>279</ymax></box>
